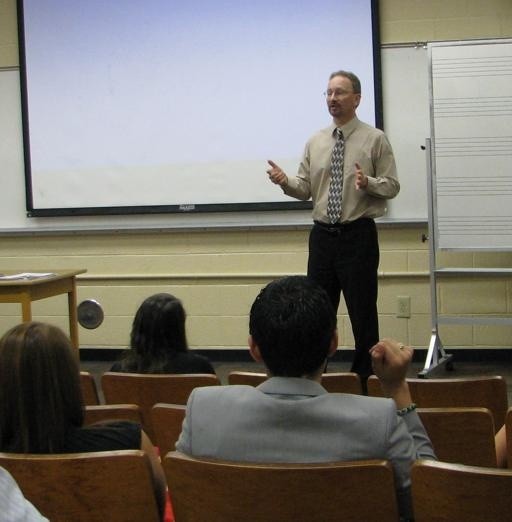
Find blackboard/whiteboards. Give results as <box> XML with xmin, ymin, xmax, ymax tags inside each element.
<box><xmin>0</xmin><ymin>42</ymin><xmax>430</xmax><ymax>234</ymax></box>
<box><xmin>429</xmin><ymin>38</ymin><xmax>512</xmax><ymax>250</ymax></box>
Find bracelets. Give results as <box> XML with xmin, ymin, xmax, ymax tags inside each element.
<box><xmin>395</xmin><ymin>403</ymin><xmax>419</xmax><ymax>418</ymax></box>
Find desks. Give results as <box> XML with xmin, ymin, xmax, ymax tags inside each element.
<box><xmin>0</xmin><ymin>263</ymin><xmax>88</xmax><ymax>369</ymax></box>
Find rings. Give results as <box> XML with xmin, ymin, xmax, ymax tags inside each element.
<box><xmin>398</xmin><ymin>342</ymin><xmax>405</xmax><ymax>349</ymax></box>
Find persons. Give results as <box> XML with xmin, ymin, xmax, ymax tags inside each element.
<box><xmin>108</xmin><ymin>291</ymin><xmax>217</xmax><ymax>378</ymax></box>
<box><xmin>174</xmin><ymin>272</ymin><xmax>441</xmax><ymax>464</ymax></box>
<box><xmin>0</xmin><ymin>320</ymin><xmax>146</xmax><ymax>459</ymax></box>
<box><xmin>1</xmin><ymin>463</ymin><xmax>56</xmax><ymax>522</ymax></box>
<box><xmin>494</xmin><ymin>411</ymin><xmax>512</xmax><ymax>469</ymax></box>
<box><xmin>266</xmin><ymin>69</ymin><xmax>401</xmax><ymax>374</ymax></box>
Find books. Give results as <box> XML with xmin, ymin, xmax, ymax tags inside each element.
<box><xmin>16</xmin><ymin>269</ymin><xmax>61</xmax><ymax>281</ymax></box>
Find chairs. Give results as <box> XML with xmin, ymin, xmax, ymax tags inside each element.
<box><xmin>0</xmin><ymin>367</ymin><xmax>512</xmax><ymax>522</ymax></box>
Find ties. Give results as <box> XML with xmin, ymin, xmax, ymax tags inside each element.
<box><xmin>327</xmin><ymin>128</ymin><xmax>345</xmax><ymax>225</ymax></box>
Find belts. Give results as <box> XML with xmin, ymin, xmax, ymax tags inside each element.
<box><xmin>314</xmin><ymin>218</ymin><xmax>371</xmax><ymax>235</ymax></box>
